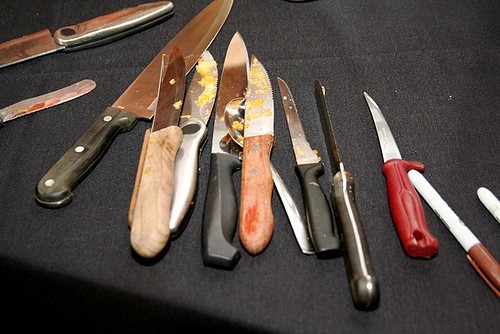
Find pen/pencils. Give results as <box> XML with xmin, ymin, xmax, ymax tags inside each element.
<box><xmin>476</xmin><ymin>187</ymin><xmax>500</xmax><ymax>223</ymax></box>
<box><xmin>408</xmin><ymin>169</ymin><xmax>500</xmax><ymax>297</ymax></box>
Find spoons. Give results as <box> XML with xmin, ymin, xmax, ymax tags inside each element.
<box><xmin>224</xmin><ymin>98</ymin><xmax>317</xmax><ymax>254</ymax></box>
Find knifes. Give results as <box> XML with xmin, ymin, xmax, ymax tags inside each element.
<box><xmin>34</xmin><ymin>0</ymin><xmax>233</xmax><ymax>209</ymax></box>
<box><xmin>0</xmin><ymin>0</ymin><xmax>177</xmax><ymax>68</ymax></box>
<box><xmin>168</xmin><ymin>50</ymin><xmax>218</xmax><ymax>233</ymax></box>
<box><xmin>128</xmin><ymin>44</ymin><xmax>187</xmax><ymax>259</ymax></box>
<box><xmin>277</xmin><ymin>77</ymin><xmax>342</xmax><ymax>257</ymax></box>
<box><xmin>239</xmin><ymin>54</ymin><xmax>275</xmax><ymax>256</ymax></box>
<box><xmin>363</xmin><ymin>91</ymin><xmax>440</xmax><ymax>259</ymax></box>
<box><xmin>313</xmin><ymin>78</ymin><xmax>381</xmax><ymax>310</ymax></box>
<box><xmin>200</xmin><ymin>30</ymin><xmax>249</xmax><ymax>272</ymax></box>
<box><xmin>0</xmin><ymin>79</ymin><xmax>97</xmax><ymax>127</ymax></box>
